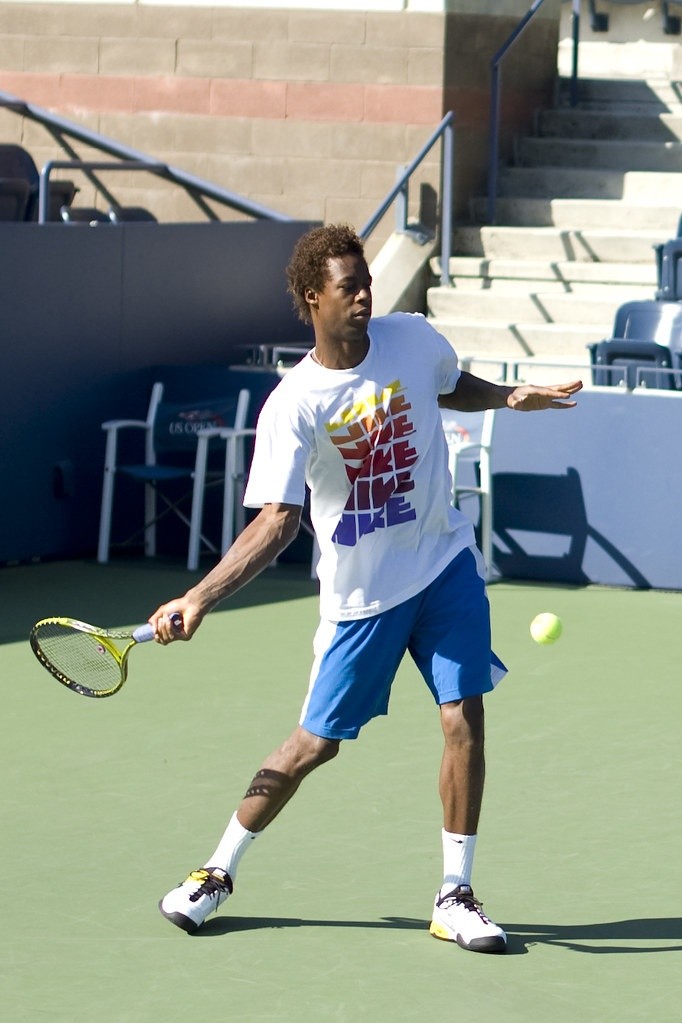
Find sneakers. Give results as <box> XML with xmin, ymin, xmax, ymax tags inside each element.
<box><xmin>430</xmin><ymin>884</ymin><xmax>507</xmax><ymax>952</ymax></box>
<box><xmin>158</xmin><ymin>867</ymin><xmax>233</xmax><ymax>935</ymax></box>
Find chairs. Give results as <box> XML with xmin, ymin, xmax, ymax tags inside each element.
<box><xmin>97</xmin><ymin>382</ymin><xmax>496</xmax><ymax>572</ymax></box>
<box><xmin>588</xmin><ymin>215</ymin><xmax>681</xmax><ymax>391</ymax></box>
<box><xmin>0</xmin><ymin>143</ymin><xmax>157</xmax><ymax>222</ymax></box>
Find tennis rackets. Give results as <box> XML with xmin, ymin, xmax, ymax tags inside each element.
<box><xmin>29</xmin><ymin>612</ymin><xmax>184</xmax><ymax>698</ymax></box>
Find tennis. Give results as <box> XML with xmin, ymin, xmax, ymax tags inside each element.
<box><xmin>530</xmin><ymin>612</ymin><xmax>562</xmax><ymax>646</ymax></box>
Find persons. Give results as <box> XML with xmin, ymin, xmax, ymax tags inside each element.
<box><xmin>147</xmin><ymin>225</ymin><xmax>583</xmax><ymax>954</ymax></box>
